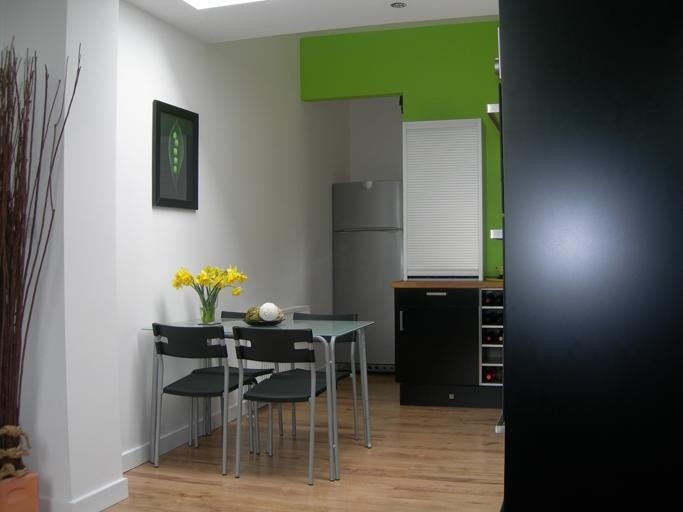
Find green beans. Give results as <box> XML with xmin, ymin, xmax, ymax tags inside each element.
<box><xmin>168</xmin><ymin>120</ymin><xmax>184</xmax><ymax>187</ymax></box>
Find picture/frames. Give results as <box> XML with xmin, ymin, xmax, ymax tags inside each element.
<box><xmin>152</xmin><ymin>100</ymin><xmax>200</xmax><ymax>210</ymax></box>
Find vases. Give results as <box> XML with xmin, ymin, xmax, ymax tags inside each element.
<box><xmin>197</xmin><ymin>307</ymin><xmax>222</xmax><ymax>324</ymax></box>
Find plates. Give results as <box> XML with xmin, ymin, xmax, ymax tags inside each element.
<box><xmin>243</xmin><ymin>319</ymin><xmax>285</xmax><ymax>326</ymax></box>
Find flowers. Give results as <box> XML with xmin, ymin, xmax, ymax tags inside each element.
<box><xmin>173</xmin><ymin>265</ymin><xmax>248</xmax><ymax>307</ymax></box>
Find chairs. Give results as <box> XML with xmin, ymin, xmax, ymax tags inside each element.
<box><xmin>149</xmin><ymin>312</ymin><xmax>375</xmax><ymax>485</ymax></box>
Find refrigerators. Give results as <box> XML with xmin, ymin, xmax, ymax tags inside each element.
<box><xmin>332</xmin><ymin>179</ymin><xmax>403</xmax><ymax>374</ymax></box>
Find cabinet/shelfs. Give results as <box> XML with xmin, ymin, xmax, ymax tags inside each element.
<box><xmin>389</xmin><ymin>280</ymin><xmax>504</xmax><ymax>408</ymax></box>
<box><xmin>485</xmin><ymin>1</ymin><xmax>683</xmax><ymax>512</ymax></box>
